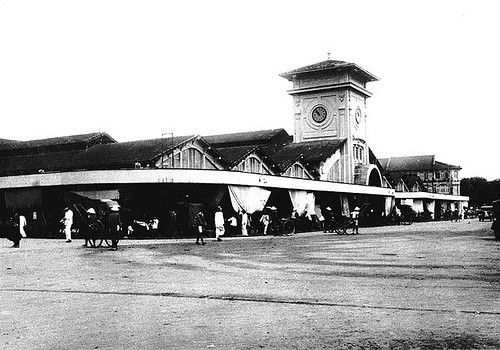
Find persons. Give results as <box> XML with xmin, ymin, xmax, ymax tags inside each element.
<box><xmin>127</xmin><ymin>223</ymin><xmax>134</xmax><ymax>239</ymax></box>
<box><xmin>109</xmin><ymin>207</ymin><xmax>122</xmax><ymax>247</ymax></box>
<box><xmin>63</xmin><ymin>206</ymin><xmax>73</xmax><ymax>242</ymax></box>
<box><xmin>83</xmin><ymin>207</ymin><xmax>96</xmax><ymax>247</ymax></box>
<box><xmin>350</xmin><ymin>207</ymin><xmax>360</xmax><ymax>234</ymax></box>
<box><xmin>9</xmin><ymin>212</ymin><xmax>27</xmax><ymax>248</ymax></box>
<box><xmin>193</xmin><ymin>210</ymin><xmax>206</xmax><ymax>245</ymax></box>
<box><xmin>440</xmin><ymin>207</ymin><xmax>492</xmax><ymax>222</ymax></box>
<box><xmin>226</xmin><ymin>204</ymin><xmax>334</xmax><ymax>236</ymax></box>
<box><xmin>215</xmin><ymin>208</ymin><xmax>225</xmax><ymax>241</ymax></box>
<box><xmin>151</xmin><ymin>216</ymin><xmax>159</xmax><ymax>239</ymax></box>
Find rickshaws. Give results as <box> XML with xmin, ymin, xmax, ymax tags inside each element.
<box><xmin>247</xmin><ymin>209</ymin><xmax>364</xmax><ymax>236</ymax></box>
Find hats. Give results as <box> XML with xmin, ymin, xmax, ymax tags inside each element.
<box><xmin>86</xmin><ymin>207</ymin><xmax>96</xmax><ymax>214</ymax></box>
<box><xmin>326</xmin><ymin>206</ymin><xmax>331</xmax><ymax>210</ymax></box>
<box><xmin>111</xmin><ymin>206</ymin><xmax>120</xmax><ymax>211</ymax></box>
<box><xmin>355</xmin><ymin>207</ymin><xmax>360</xmax><ymax>210</ymax></box>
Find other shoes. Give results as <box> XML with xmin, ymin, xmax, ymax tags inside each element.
<box><xmin>218</xmin><ymin>239</ymin><xmax>222</xmax><ymax>241</ymax></box>
<box><xmin>66</xmin><ymin>241</ymin><xmax>71</xmax><ymax>242</ymax></box>
<box><xmin>202</xmin><ymin>243</ymin><xmax>206</xmax><ymax>245</ymax></box>
<box><xmin>196</xmin><ymin>242</ymin><xmax>201</xmax><ymax>245</ymax></box>
